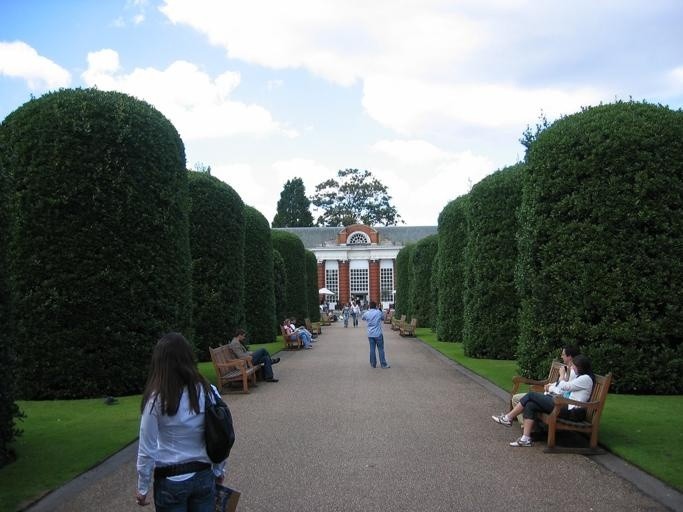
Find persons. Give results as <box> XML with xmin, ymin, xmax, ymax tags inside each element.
<box><xmin>361</xmin><ymin>301</ymin><xmax>391</xmax><ymax>369</ymax></box>
<box><xmin>135</xmin><ymin>332</ymin><xmax>228</xmax><ymax>512</ymax></box>
<box><xmin>229</xmin><ymin>328</ymin><xmax>280</xmax><ymax>382</ymax></box>
<box><xmin>491</xmin><ymin>354</ymin><xmax>596</xmax><ymax>447</ymax></box>
<box><xmin>513</xmin><ymin>344</ymin><xmax>581</xmax><ymax>433</ymax></box>
<box><xmin>281</xmin><ymin>298</ymin><xmax>361</xmax><ymax>350</ymax></box>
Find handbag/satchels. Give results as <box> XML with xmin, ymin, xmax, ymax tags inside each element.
<box><xmin>201</xmin><ymin>382</ymin><xmax>235</xmax><ymax>465</ymax></box>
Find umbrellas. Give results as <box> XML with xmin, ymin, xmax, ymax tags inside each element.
<box><xmin>319</xmin><ymin>287</ymin><xmax>335</xmax><ymax>295</ymax></box>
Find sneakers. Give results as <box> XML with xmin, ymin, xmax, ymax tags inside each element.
<box><xmin>270</xmin><ymin>358</ymin><xmax>280</xmax><ymax>365</ymax></box>
<box><xmin>491</xmin><ymin>415</ymin><xmax>513</xmax><ymax>429</ymax></box>
<box><xmin>383</xmin><ymin>365</ymin><xmax>391</xmax><ymax>369</ymax></box>
<box><xmin>508</xmin><ymin>436</ymin><xmax>532</xmax><ymax>448</ymax></box>
<box><xmin>266</xmin><ymin>378</ymin><xmax>279</xmax><ymax>382</ymax></box>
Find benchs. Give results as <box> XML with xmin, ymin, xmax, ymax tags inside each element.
<box><xmin>509</xmin><ymin>359</ymin><xmax>613</xmax><ymax>455</ymax></box>
<box><xmin>208</xmin><ymin>341</ymin><xmax>265</xmax><ymax>394</ymax></box>
<box><xmin>278</xmin><ymin>312</ymin><xmax>335</xmax><ymax>351</ymax></box>
<box><xmin>381</xmin><ymin>309</ymin><xmax>418</xmax><ymax>338</ymax></box>
